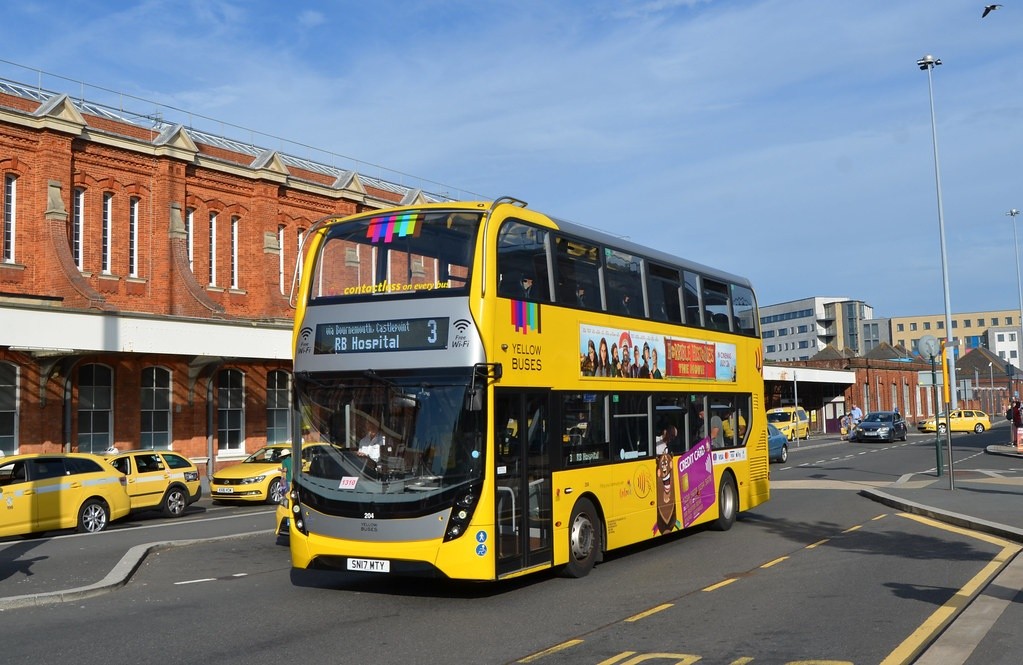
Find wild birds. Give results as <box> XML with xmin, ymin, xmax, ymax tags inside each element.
<box><xmin>981</xmin><ymin>4</ymin><xmax>1003</xmax><ymax>18</ymax></box>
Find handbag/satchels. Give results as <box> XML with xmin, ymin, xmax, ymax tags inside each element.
<box><xmin>841</xmin><ymin>426</ymin><xmax>847</xmax><ymax>434</ymax></box>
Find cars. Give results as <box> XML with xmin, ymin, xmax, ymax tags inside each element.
<box><xmin>275</xmin><ymin>461</ymin><xmax>313</xmax><ymax>534</ymax></box>
<box><xmin>102</xmin><ymin>448</ymin><xmax>203</xmax><ymax>519</ymax></box>
<box><xmin>851</xmin><ymin>412</ymin><xmax>909</xmax><ymax>444</ymax></box>
<box><xmin>0</xmin><ymin>450</ymin><xmax>131</xmax><ymax>538</ymax></box>
<box><xmin>209</xmin><ymin>443</ymin><xmax>343</xmax><ymax>504</ymax></box>
<box><xmin>767</xmin><ymin>423</ymin><xmax>793</xmax><ymax>461</ymax></box>
<box><xmin>918</xmin><ymin>409</ymin><xmax>991</xmax><ymax>434</ymax></box>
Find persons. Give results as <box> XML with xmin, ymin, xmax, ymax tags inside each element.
<box><xmin>581</xmin><ymin>338</ymin><xmax>662</xmax><ymax>379</ymax></box>
<box><xmin>1012</xmin><ymin>402</ymin><xmax>1023</xmax><ymax>446</ymax></box>
<box><xmin>839</xmin><ymin>404</ymin><xmax>863</xmax><ymax>441</ymax></box>
<box><xmin>278</xmin><ymin>449</ymin><xmax>292</xmax><ymax>491</ymax></box>
<box><xmin>359</xmin><ymin>416</ymin><xmax>393</xmax><ymax>472</ymax></box>
<box><xmin>565</xmin><ymin>404</ymin><xmax>746</xmax><ymax>462</ymax></box>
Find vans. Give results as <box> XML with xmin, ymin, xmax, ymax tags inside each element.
<box><xmin>766</xmin><ymin>407</ymin><xmax>810</xmax><ymax>439</ymax></box>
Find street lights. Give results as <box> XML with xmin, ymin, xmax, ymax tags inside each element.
<box><xmin>918</xmin><ymin>50</ymin><xmax>970</xmax><ymax>481</ymax></box>
<box><xmin>1004</xmin><ymin>209</ymin><xmax>1022</xmax><ymax>325</ymax></box>
<box><xmin>987</xmin><ymin>361</ymin><xmax>997</xmax><ymax>421</ymax></box>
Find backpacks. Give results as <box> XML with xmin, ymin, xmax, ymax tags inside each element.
<box><xmin>1006</xmin><ymin>408</ymin><xmax>1014</xmax><ymax>421</ymax></box>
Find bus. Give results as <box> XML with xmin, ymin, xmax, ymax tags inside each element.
<box><xmin>286</xmin><ymin>194</ymin><xmax>774</xmax><ymax>584</ymax></box>
